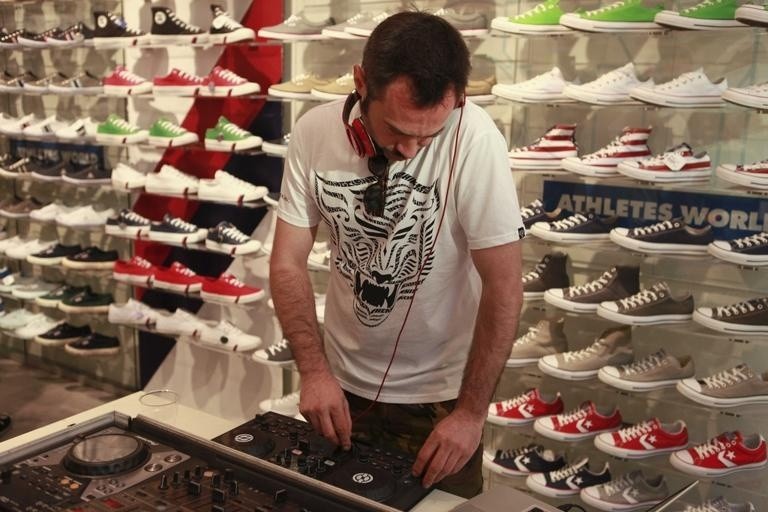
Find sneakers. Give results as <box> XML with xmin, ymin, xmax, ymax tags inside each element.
<box><xmin>562</xmin><ymin>62</ymin><xmax>657</xmax><ymax>108</ymax></box>
<box><xmin>462</xmin><ymin>74</ymin><xmax>499</xmax><ymax>105</ymax></box>
<box><xmin>0</xmin><ymin>1</ymin><xmax>421</xmax><ymax>367</ymax></box>
<box><xmin>491</xmin><ymin>66</ymin><xmax>583</xmax><ymax>105</ymax></box>
<box><xmin>616</xmin><ymin>142</ymin><xmax>712</xmax><ymax>184</ymax></box>
<box><xmin>654</xmin><ymin>0</ymin><xmax>758</xmax><ymax>33</ymax></box>
<box><xmin>721</xmin><ymin>80</ymin><xmax>768</xmax><ymax>112</ymax></box>
<box><xmin>733</xmin><ymin>1</ymin><xmax>767</xmax><ymax>29</ymax></box>
<box><xmin>715</xmin><ymin>155</ymin><xmax>768</xmax><ymax>191</ymax></box>
<box><xmin>507</xmin><ymin>120</ymin><xmax>581</xmax><ymax>173</ymax></box>
<box><xmin>558</xmin><ymin>0</ymin><xmax>672</xmax><ymax>36</ymax></box>
<box><xmin>490</xmin><ymin>0</ymin><xmax>577</xmax><ymax>37</ymax></box>
<box><xmin>431</xmin><ymin>6</ymin><xmax>490</xmax><ymax>39</ymax></box>
<box><xmin>561</xmin><ymin>125</ymin><xmax>655</xmax><ymax>180</ymax></box>
<box><xmin>627</xmin><ymin>64</ymin><xmax>729</xmax><ymax>110</ymax></box>
<box><xmin>481</xmin><ymin>199</ymin><xmax>767</xmax><ymax>512</ymax></box>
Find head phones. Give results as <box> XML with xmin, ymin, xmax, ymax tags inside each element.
<box><xmin>343</xmin><ymin>86</ymin><xmax>466</xmax><ymax>158</ymax></box>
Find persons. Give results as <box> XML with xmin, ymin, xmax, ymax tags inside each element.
<box><xmin>268</xmin><ymin>2</ymin><xmax>526</xmax><ymax>502</ymax></box>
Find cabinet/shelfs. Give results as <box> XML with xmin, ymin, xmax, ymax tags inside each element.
<box><xmin>0</xmin><ymin>1</ymin><xmax>767</xmax><ymax>511</ymax></box>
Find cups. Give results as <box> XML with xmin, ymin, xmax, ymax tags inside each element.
<box><xmin>137</xmin><ymin>390</ymin><xmax>179</xmax><ymax>430</ymax></box>
